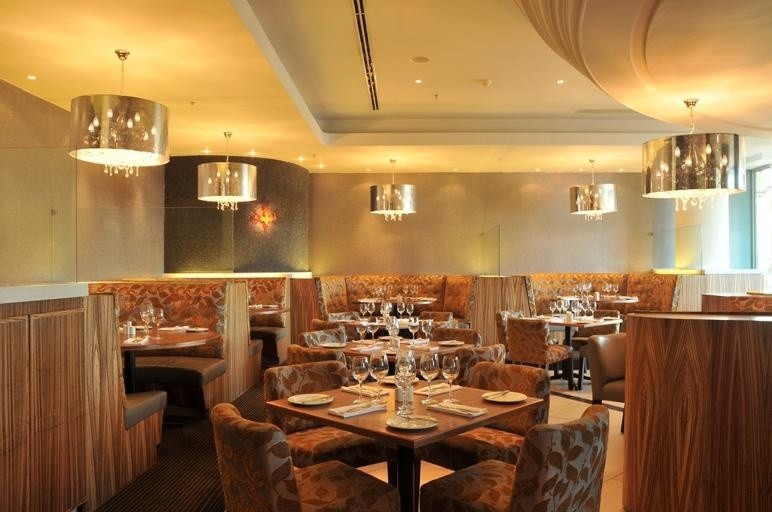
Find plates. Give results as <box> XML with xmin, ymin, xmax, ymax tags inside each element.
<box><xmin>263</xmin><ymin>304</ymin><xmax>278</xmax><ymax>309</ymax></box>
<box><xmin>288</xmin><ymin>392</ymin><xmax>334</xmax><ymax>406</ymax></box>
<box><xmin>318</xmin><ymin>343</ymin><xmax>347</xmax><ymax>348</ymax></box>
<box><xmin>482</xmin><ymin>391</ymin><xmax>526</xmax><ymax>402</ymax></box>
<box><xmin>386</xmin><ymin>414</ymin><xmax>439</xmax><ymax>429</ymax></box>
<box><xmin>187</xmin><ymin>327</ymin><xmax>208</xmax><ymax>332</ymax></box>
<box><xmin>439</xmin><ymin>341</ymin><xmax>464</xmax><ymax>346</ymax></box>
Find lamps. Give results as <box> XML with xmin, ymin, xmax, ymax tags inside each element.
<box><xmin>198</xmin><ymin>129</ymin><xmax>258</xmax><ymax>211</ymax></box>
<box><xmin>370</xmin><ymin>159</ymin><xmax>416</xmax><ymax>224</ymax></box>
<box><xmin>642</xmin><ymin>96</ymin><xmax>746</xmax><ymax>210</ymax></box>
<box><xmin>69</xmin><ymin>50</ymin><xmax>168</xmax><ymax>177</ymax></box>
<box><xmin>569</xmin><ymin>159</ymin><xmax>617</xmax><ymax>220</ymax></box>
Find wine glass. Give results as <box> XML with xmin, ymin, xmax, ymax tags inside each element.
<box><xmin>153</xmin><ymin>308</ymin><xmax>165</xmax><ymax>339</ymax></box>
<box><xmin>356</xmin><ymin>284</ymin><xmax>432</xmax><ymax>352</ymax></box>
<box><xmin>140</xmin><ymin>310</ymin><xmax>153</xmax><ymax>335</ymax></box>
<box><xmin>549</xmin><ymin>282</ymin><xmax>619</xmax><ymax>319</ymax></box>
<box><xmin>353</xmin><ymin>353</ymin><xmax>460</xmax><ymax>414</ymax></box>
